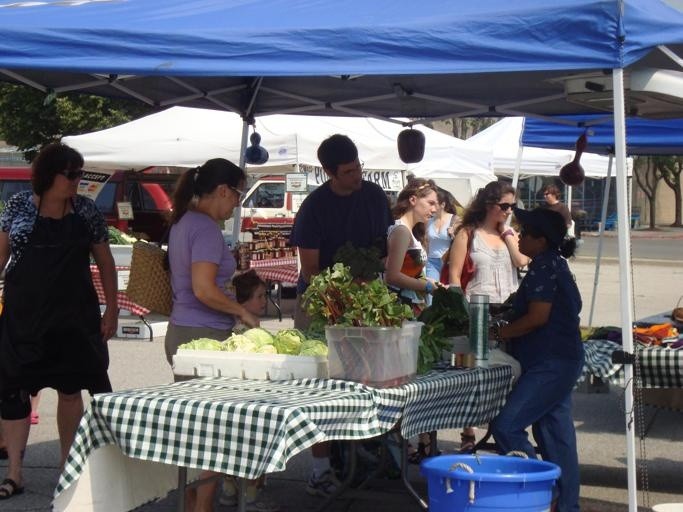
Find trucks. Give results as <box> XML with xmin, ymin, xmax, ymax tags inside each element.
<box><xmin>241</xmin><ymin>175</ymin><xmax>298</xmax><ymax>235</ymax></box>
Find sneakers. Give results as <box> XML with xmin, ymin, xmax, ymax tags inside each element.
<box><xmin>306</xmin><ymin>470</ymin><xmax>354</xmax><ymax>500</ymax></box>
<box><xmin>220</xmin><ymin>487</ymin><xmax>281</xmax><ymax>512</ymax></box>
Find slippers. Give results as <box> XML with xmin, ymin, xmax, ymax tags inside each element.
<box><xmin>0</xmin><ymin>479</ymin><xmax>25</xmax><ymax>499</ymax></box>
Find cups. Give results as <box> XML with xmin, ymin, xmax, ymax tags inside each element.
<box><xmin>470</xmin><ymin>294</ymin><xmax>490</xmax><ymax>360</ymax></box>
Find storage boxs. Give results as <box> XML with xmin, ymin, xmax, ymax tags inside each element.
<box><xmin>116</xmin><ymin>317</ymin><xmax>169</xmax><ymax>339</ymax></box>
<box><xmin>326</xmin><ymin>322</ymin><xmax>423</xmax><ymax>388</ymax></box>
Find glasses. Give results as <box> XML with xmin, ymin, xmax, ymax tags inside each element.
<box><xmin>59</xmin><ymin>170</ymin><xmax>84</xmax><ymax>178</ymax></box>
<box><xmin>337</xmin><ymin>160</ymin><xmax>364</xmax><ymax>176</ymax></box>
<box><xmin>493</xmin><ymin>203</ymin><xmax>517</xmax><ymax>210</ymax></box>
<box><xmin>229</xmin><ymin>187</ymin><xmax>246</xmax><ymax>202</ymax></box>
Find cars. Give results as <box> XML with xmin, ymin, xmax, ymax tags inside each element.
<box><xmin>0</xmin><ymin>167</ymin><xmax>188</xmax><ymax>244</ymax></box>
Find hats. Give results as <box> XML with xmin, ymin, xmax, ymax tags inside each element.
<box><xmin>513</xmin><ymin>207</ymin><xmax>567</xmax><ymax>244</ymax></box>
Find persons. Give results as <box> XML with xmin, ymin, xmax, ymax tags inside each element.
<box><xmin>485</xmin><ymin>207</ymin><xmax>588</xmax><ymax>510</ymax></box>
<box><xmin>543</xmin><ymin>183</ymin><xmax>572</xmax><ymax>230</ymax></box>
<box><xmin>290</xmin><ymin>133</ymin><xmax>394</xmax><ymax>499</ymax></box>
<box><xmin>220</xmin><ymin>269</ymin><xmax>278</xmax><ymax>510</ymax></box>
<box><xmin>162</xmin><ymin>157</ymin><xmax>259</xmax><ymax>511</ymax></box>
<box><xmin>0</xmin><ymin>143</ymin><xmax>122</xmax><ymax>500</ymax></box>
<box><xmin>388</xmin><ymin>178</ymin><xmax>531</xmax><ymax>464</ymax></box>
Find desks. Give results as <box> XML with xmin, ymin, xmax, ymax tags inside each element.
<box><xmin>250</xmin><ymin>259</ymin><xmax>300</xmax><ymax>321</ymax></box>
<box><xmin>609</xmin><ymin>309</ymin><xmax>683</xmax><ymax>439</ymax></box>
<box><xmin>52</xmin><ymin>338</ymin><xmax>623</xmax><ymax>511</ymax></box>
<box><xmin>90</xmin><ymin>261</ymin><xmax>153</xmax><ymax>342</ymax></box>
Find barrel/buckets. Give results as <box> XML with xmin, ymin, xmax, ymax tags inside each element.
<box><xmin>416</xmin><ymin>450</ymin><xmax>562</xmax><ymax>512</ymax></box>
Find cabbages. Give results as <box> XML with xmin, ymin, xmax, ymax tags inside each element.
<box><xmin>179</xmin><ymin>327</ymin><xmax>326</xmax><ymax>358</ymax></box>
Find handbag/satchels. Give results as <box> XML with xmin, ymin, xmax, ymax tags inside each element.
<box><xmin>126</xmin><ymin>240</ymin><xmax>173</xmax><ymax>316</ymax></box>
<box><xmin>400</xmin><ymin>249</ymin><xmax>423</xmax><ymax>277</ymax></box>
<box><xmin>439</xmin><ymin>249</ymin><xmax>475</xmax><ymax>295</ymax></box>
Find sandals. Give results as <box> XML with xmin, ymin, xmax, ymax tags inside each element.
<box><xmin>408</xmin><ymin>433</ymin><xmax>476</xmax><ymax>465</ymax></box>
<box><xmin>31</xmin><ymin>413</ymin><xmax>39</xmax><ymax>423</ymax></box>
